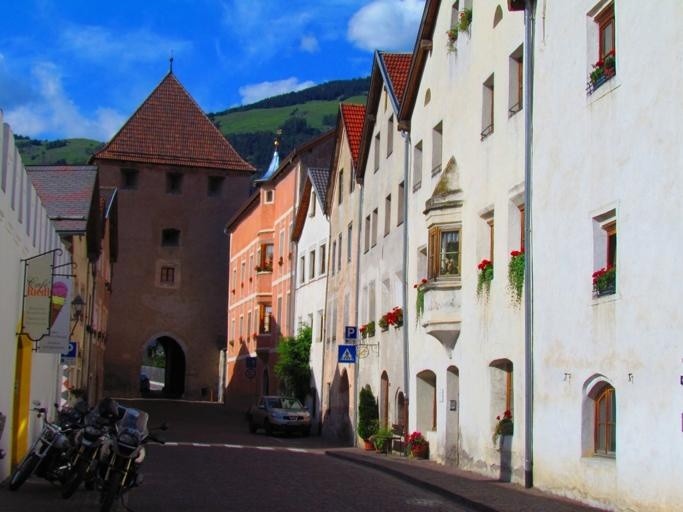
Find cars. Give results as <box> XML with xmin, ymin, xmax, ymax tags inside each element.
<box><xmin>140</xmin><ymin>373</ymin><xmax>150</xmax><ymax>393</ymax></box>
<box><xmin>245</xmin><ymin>395</ymin><xmax>311</xmax><ymax>437</ymax></box>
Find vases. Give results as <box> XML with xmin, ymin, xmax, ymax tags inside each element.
<box><xmin>413</xmin><ymin>449</ymin><xmax>427</xmax><ymax>460</ymax></box>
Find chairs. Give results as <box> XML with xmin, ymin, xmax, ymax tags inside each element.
<box><xmin>379</xmin><ymin>423</ymin><xmax>405</xmax><ymax>457</ymax></box>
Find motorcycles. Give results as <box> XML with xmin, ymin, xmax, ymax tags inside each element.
<box><xmin>7</xmin><ymin>396</ymin><xmax>169</xmax><ymax>511</ymax></box>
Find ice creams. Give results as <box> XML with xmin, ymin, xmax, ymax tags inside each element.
<box><xmin>50</xmin><ymin>282</ymin><xmax>67</xmax><ymax>328</ymax></box>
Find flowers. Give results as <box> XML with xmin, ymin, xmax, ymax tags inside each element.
<box><xmin>409</xmin><ymin>430</ymin><xmax>425</xmax><ymax>452</ymax></box>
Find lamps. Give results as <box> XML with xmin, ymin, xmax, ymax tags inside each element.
<box><xmin>69</xmin><ymin>295</ymin><xmax>86</xmax><ymax>340</ymax></box>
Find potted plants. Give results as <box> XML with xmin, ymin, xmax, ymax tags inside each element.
<box><xmin>358</xmin><ymin>384</ymin><xmax>379</xmax><ymax>452</ymax></box>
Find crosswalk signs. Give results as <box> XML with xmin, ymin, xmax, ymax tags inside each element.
<box><xmin>337</xmin><ymin>344</ymin><xmax>356</xmax><ymax>364</ymax></box>
